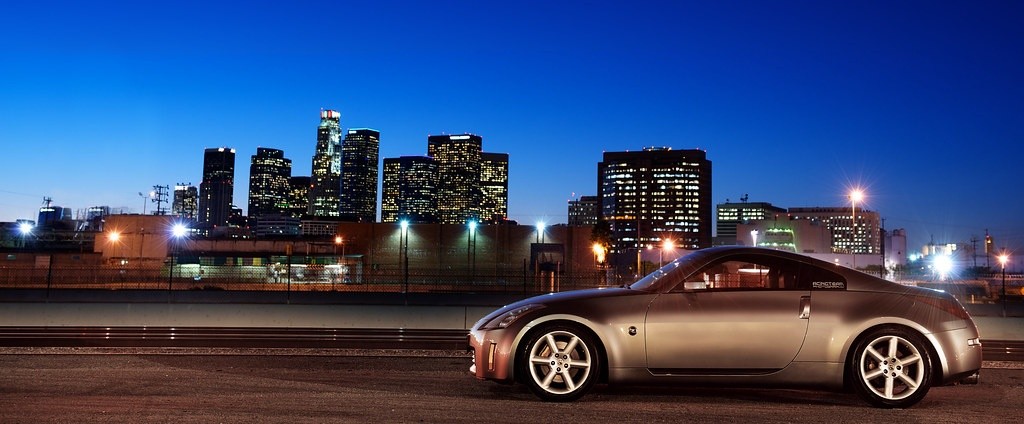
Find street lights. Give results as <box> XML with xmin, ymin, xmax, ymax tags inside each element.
<box><xmin>336</xmin><ymin>238</ymin><xmax>344</xmax><ymax>283</ymax></box>
<box><xmin>538</xmin><ymin>223</ymin><xmax>545</xmax><ymax>265</ymax></box>
<box><xmin>660</xmin><ymin>242</ymin><xmax>673</xmax><ymax>268</ymax></box>
<box><xmin>469</xmin><ymin>221</ymin><xmax>477</xmax><ymax>280</ymax></box>
<box><xmin>999</xmin><ymin>255</ymin><xmax>1008</xmax><ymax>318</ymax></box>
<box><xmin>110</xmin><ymin>192</ymin><xmax>185</xmax><ymax>305</ymax></box>
<box><xmin>852</xmin><ymin>191</ymin><xmax>862</xmax><ymax>270</ymax></box>
<box><xmin>593</xmin><ymin>242</ymin><xmax>602</xmax><ymax>266</ymax></box>
<box><xmin>401</xmin><ymin>221</ymin><xmax>409</xmax><ymax>293</ymax></box>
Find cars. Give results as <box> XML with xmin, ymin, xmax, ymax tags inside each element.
<box><xmin>467</xmin><ymin>244</ymin><xmax>983</xmax><ymax>409</ymax></box>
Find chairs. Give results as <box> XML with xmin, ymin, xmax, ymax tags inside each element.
<box><xmin>766</xmin><ymin>261</ymin><xmax>795</xmax><ymax>289</ymax></box>
